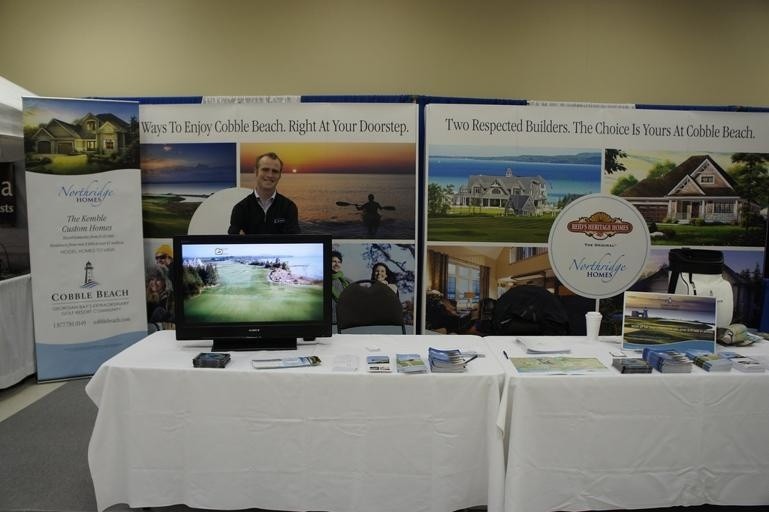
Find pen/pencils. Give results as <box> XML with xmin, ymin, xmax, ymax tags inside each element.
<box><xmin>503</xmin><ymin>350</ymin><xmax>509</xmax><ymax>360</ymax></box>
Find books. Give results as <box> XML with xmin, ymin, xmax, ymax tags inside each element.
<box><xmin>396</xmin><ymin>352</ymin><xmax>425</xmax><ymax>373</ymax></box>
<box><xmin>194</xmin><ymin>353</ymin><xmax>230</xmax><ymax>368</ymax></box>
<box><xmin>607</xmin><ymin>351</ymin><xmax>652</xmax><ymax>373</ymax></box>
<box><xmin>518</xmin><ymin>337</ymin><xmax>572</xmax><ymax>354</ymax></box>
<box><xmin>642</xmin><ymin>347</ymin><xmax>693</xmax><ymax>373</ymax></box>
<box><xmin>367</xmin><ymin>354</ymin><xmax>391</xmax><ymax>373</ymax></box>
<box><xmin>253</xmin><ymin>356</ymin><xmax>321</xmax><ymax>370</ymax></box>
<box><xmin>429</xmin><ymin>347</ymin><xmax>467</xmax><ymax>374</ymax></box>
<box><xmin>721</xmin><ymin>351</ymin><xmax>764</xmax><ymax>372</ymax></box>
<box><xmin>687</xmin><ymin>349</ymin><xmax>731</xmax><ymax>372</ymax></box>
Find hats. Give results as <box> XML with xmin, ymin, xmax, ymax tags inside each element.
<box><xmin>154</xmin><ymin>244</ymin><xmax>174</xmax><ymax>258</ymax></box>
<box><xmin>146</xmin><ymin>263</ymin><xmax>169</xmax><ymax>279</ymax></box>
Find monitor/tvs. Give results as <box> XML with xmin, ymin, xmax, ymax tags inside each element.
<box><xmin>171</xmin><ymin>234</ymin><xmax>332</xmax><ymax>352</ymax></box>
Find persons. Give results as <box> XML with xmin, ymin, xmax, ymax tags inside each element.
<box><xmin>373</xmin><ymin>263</ymin><xmax>398</xmax><ymax>294</ymax></box>
<box><xmin>154</xmin><ymin>244</ymin><xmax>173</xmax><ymax>287</ymax></box>
<box><xmin>145</xmin><ymin>263</ymin><xmax>175</xmax><ymax>335</ymax></box>
<box><xmin>355</xmin><ymin>194</ymin><xmax>383</xmax><ymax>223</ymax></box>
<box><xmin>229</xmin><ymin>152</ymin><xmax>300</xmax><ymax>235</ymax></box>
<box><xmin>331</xmin><ymin>251</ymin><xmax>354</xmax><ymax>326</ymax></box>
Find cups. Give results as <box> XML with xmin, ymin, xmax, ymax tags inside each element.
<box><xmin>586</xmin><ymin>312</ymin><xmax>602</xmax><ymax>339</ymax></box>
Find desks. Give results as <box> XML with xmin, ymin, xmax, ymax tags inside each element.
<box><xmin>87</xmin><ymin>330</ymin><xmax>504</xmax><ymax>511</ymax></box>
<box><xmin>484</xmin><ymin>334</ymin><xmax>768</xmax><ymax>512</ymax></box>
